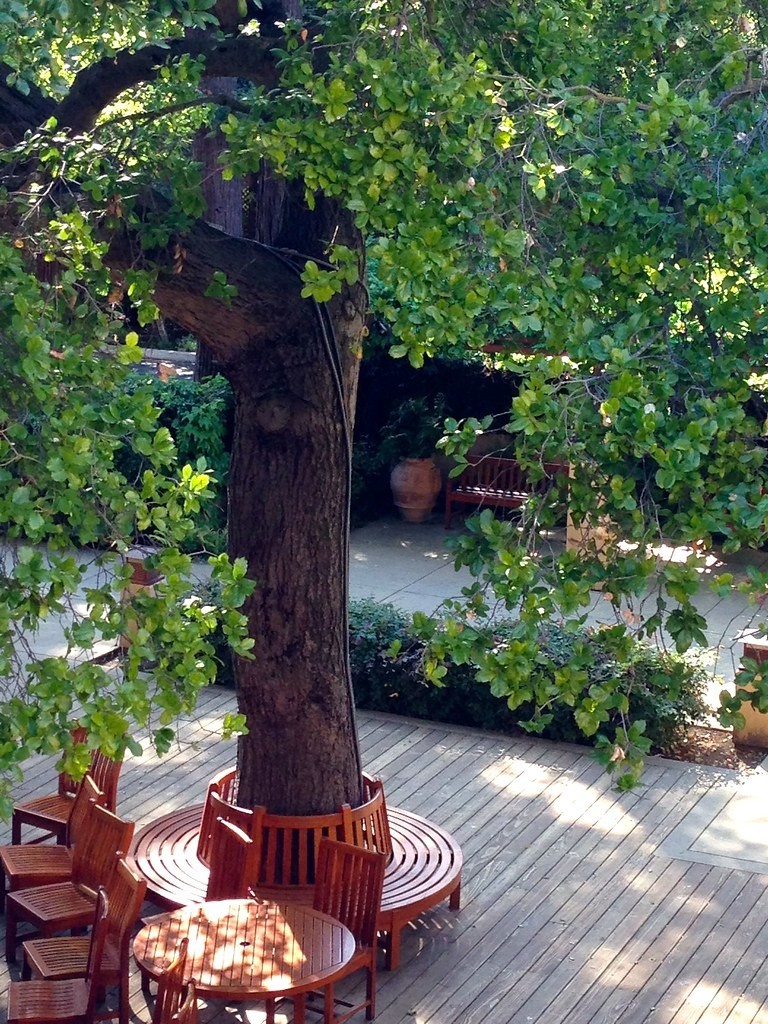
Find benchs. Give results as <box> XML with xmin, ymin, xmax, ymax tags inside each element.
<box><xmin>443</xmin><ymin>449</ymin><xmax>569</xmax><ymax>532</ymax></box>
<box><xmin>129</xmin><ymin>762</ymin><xmax>466</xmax><ymax>972</ymax></box>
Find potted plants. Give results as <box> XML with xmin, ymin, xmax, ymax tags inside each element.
<box><xmin>379</xmin><ymin>392</ymin><xmax>453</xmax><ymax>524</ymax></box>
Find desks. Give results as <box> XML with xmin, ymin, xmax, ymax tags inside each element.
<box><xmin>133</xmin><ymin>898</ymin><xmax>357</xmax><ymax>1024</ymax></box>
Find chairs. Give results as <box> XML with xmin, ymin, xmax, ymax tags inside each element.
<box><xmin>0</xmin><ymin>718</ymin><xmax>389</xmax><ymax>1024</ymax></box>
